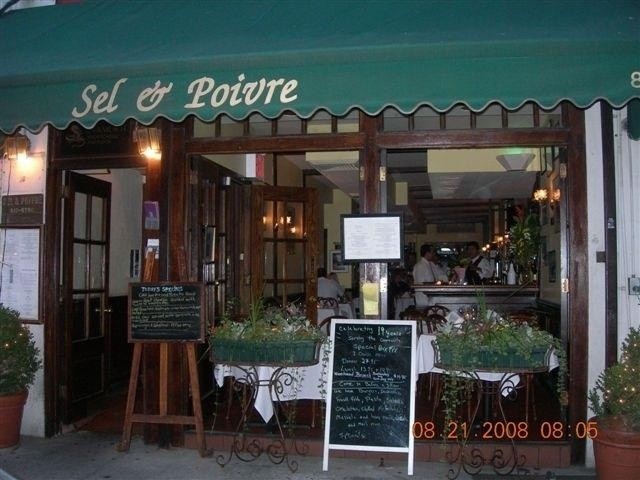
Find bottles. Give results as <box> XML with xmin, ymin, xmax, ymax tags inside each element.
<box><xmin>507</xmin><ymin>262</ymin><xmax>517</xmax><ymax>286</ymax></box>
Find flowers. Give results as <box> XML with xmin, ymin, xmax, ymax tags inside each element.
<box><xmin>505</xmin><ymin>203</ymin><xmax>541</xmax><ymax>264</ymax></box>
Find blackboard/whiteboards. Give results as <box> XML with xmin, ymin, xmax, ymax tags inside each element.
<box><xmin>128</xmin><ymin>282</ymin><xmax>204</xmax><ymax>343</ymax></box>
<box><xmin>324</xmin><ymin>319</ymin><xmax>418</xmax><ymax>452</ymax></box>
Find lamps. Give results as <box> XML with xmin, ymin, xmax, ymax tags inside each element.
<box><xmin>7</xmin><ymin>134</ymin><xmax>27</xmax><ymax>162</ymax></box>
<box><xmin>134</xmin><ymin>125</ymin><xmax>160</xmax><ymax>159</ymax></box>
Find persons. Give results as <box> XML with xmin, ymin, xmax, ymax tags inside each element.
<box><xmin>315</xmin><ymin>240</ymin><xmax>494</xmax><ymax>336</ymax></box>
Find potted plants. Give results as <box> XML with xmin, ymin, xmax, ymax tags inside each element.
<box><xmin>586</xmin><ymin>323</ymin><xmax>640</xmax><ymax>480</ymax></box>
<box><xmin>434</xmin><ymin>280</ymin><xmax>567</xmax><ymax>372</ymax></box>
<box><xmin>1</xmin><ymin>304</ymin><xmax>45</xmax><ymax>448</ymax></box>
<box><xmin>207</xmin><ymin>296</ymin><xmax>331</xmax><ymax>366</ymax></box>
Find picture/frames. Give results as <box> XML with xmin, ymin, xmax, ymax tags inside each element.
<box><xmin>329</xmin><ymin>250</ymin><xmax>349</xmax><ymax>273</ymax></box>
<box><xmin>339</xmin><ymin>211</ymin><xmax>406</xmax><ymax>262</ymax></box>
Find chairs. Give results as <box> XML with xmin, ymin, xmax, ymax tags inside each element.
<box><xmin>317</xmin><ymin>296</ymin><xmax>339</xmax><ymax>308</ymax></box>
<box><xmin>400</xmin><ymin>304</ymin><xmax>450</xmax><ymax>336</ymax></box>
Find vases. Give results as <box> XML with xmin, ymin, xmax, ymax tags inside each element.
<box><xmin>522</xmin><ymin>265</ymin><xmax>534</xmax><ymax>284</ymax></box>
